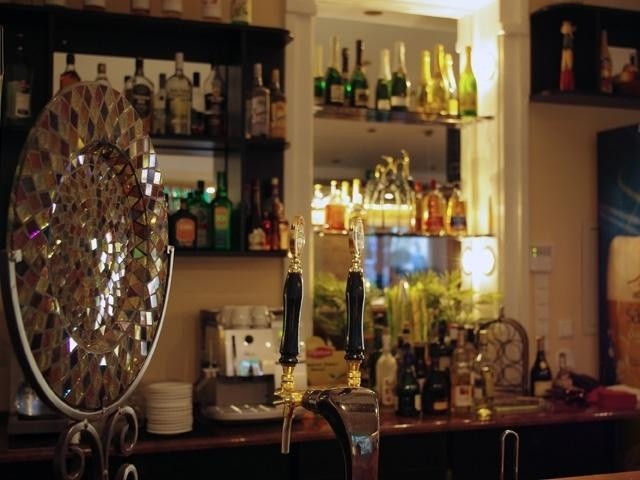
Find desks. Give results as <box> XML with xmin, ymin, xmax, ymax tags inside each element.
<box><xmin>0</xmin><ymin>391</ymin><xmax>640</xmax><ymax>480</ymax></box>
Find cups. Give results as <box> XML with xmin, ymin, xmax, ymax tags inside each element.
<box><xmin>216</xmin><ymin>305</ymin><xmax>277</xmax><ymax>328</ymax></box>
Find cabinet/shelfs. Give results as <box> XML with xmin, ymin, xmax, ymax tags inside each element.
<box><xmin>314</xmin><ymin>104</ymin><xmax>494</xmax><ymax>350</ymax></box>
<box><xmin>0</xmin><ymin>1</ymin><xmax>293</xmax><ymax>257</ymax></box>
<box><xmin>531</xmin><ymin>3</ymin><xmax>639</xmax><ymax>112</ymax></box>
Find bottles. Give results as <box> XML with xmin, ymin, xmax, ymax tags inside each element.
<box><xmin>601</xmin><ymin>30</ymin><xmax>613</xmax><ymax>94</ymax></box>
<box><xmin>411</xmin><ymin>178</ymin><xmax>468</xmax><ymax>236</ymax></box>
<box><xmin>147</xmin><ymin>382</ymin><xmax>194</xmax><ymax>434</ymax></box>
<box><xmin>311</xmin><ymin>177</ymin><xmax>362</xmax><ymax>233</ymax></box>
<box><xmin>164</xmin><ymin>171</ymin><xmax>289</xmax><ymax>252</ymax></box>
<box><xmin>312</xmin><ymin>35</ymin><xmax>478</xmax><ymax>117</ymax></box>
<box><xmin>2</xmin><ymin>33</ymin><xmax>288</xmax><ymax>140</ymax></box>
<box><xmin>529</xmin><ymin>336</ymin><xmax>554</xmax><ymax>399</ymax></box>
<box><xmin>368</xmin><ymin>312</ymin><xmax>497</xmax><ymax>424</ymax></box>
<box><xmin>559</xmin><ymin>21</ymin><xmax>579</xmax><ymax>91</ymax></box>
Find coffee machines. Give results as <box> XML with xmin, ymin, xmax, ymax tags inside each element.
<box><xmin>195</xmin><ymin>308</ymin><xmax>308</xmax><ymax>423</ymax></box>
